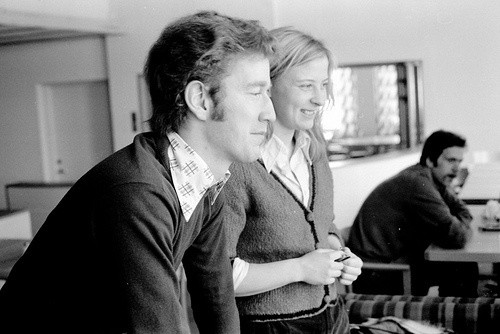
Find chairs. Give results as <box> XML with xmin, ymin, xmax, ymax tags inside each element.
<box><xmin>340</xmin><ymin>226</ymin><xmax>413</xmax><ymax>296</ymax></box>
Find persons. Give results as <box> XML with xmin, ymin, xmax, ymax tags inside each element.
<box><xmin>348</xmin><ymin>131</ymin><xmax>479</xmax><ymax>297</ymax></box>
<box><xmin>0</xmin><ymin>12</ymin><xmax>276</xmax><ymax>334</ymax></box>
<box><xmin>216</xmin><ymin>27</ymin><xmax>363</xmax><ymax>334</ymax></box>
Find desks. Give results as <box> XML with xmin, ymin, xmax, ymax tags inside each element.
<box><xmin>424</xmin><ymin>205</ymin><xmax>500</xmax><ymax>262</ymax></box>
